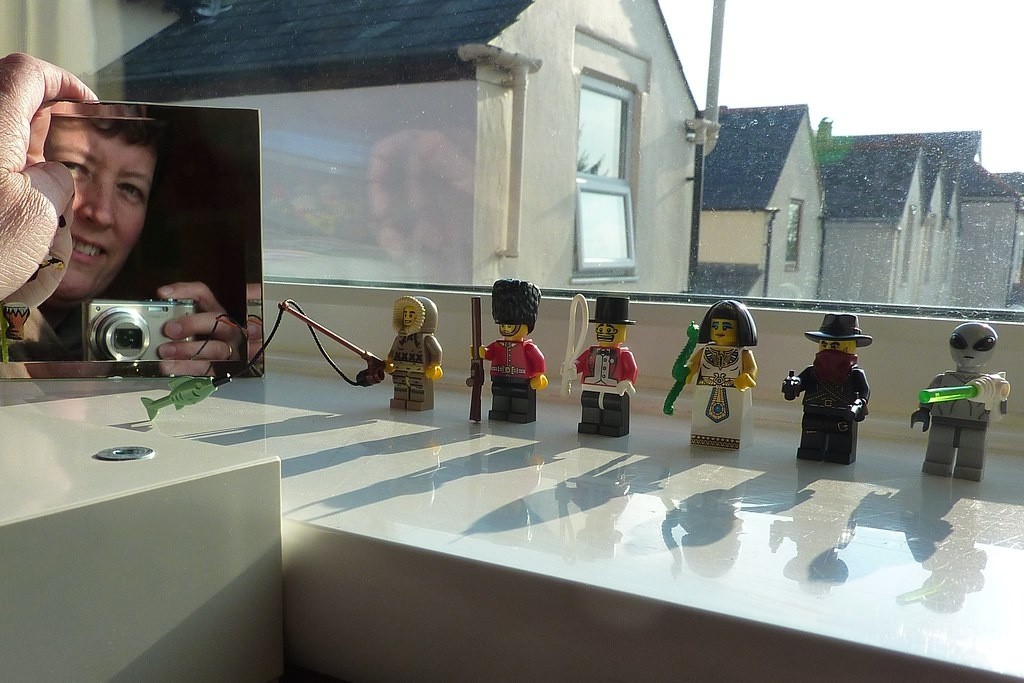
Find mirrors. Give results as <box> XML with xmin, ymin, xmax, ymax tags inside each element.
<box><xmin>0</xmin><ymin>99</ymin><xmax>264</xmax><ymax>379</ymax></box>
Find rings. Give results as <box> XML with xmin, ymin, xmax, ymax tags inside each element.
<box><xmin>227</xmin><ymin>343</ymin><xmax>232</xmax><ymax>356</ymax></box>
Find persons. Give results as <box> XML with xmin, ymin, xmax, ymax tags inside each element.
<box><xmin>384</xmin><ymin>295</ymin><xmax>444</xmax><ymax>411</ymax></box>
<box><xmin>0</xmin><ymin>52</ymin><xmax>99</xmax><ymax>303</ymax></box>
<box><xmin>782</xmin><ymin>312</ymin><xmax>872</xmax><ymax>465</ymax></box>
<box><xmin>684</xmin><ymin>300</ymin><xmax>758</xmax><ymax>450</ymax></box>
<box><xmin>911</xmin><ymin>323</ymin><xmax>1009</xmax><ymax>482</ymax></box>
<box><xmin>469</xmin><ymin>278</ymin><xmax>548</xmax><ymax>424</ymax></box>
<box><xmin>560</xmin><ymin>296</ymin><xmax>638</xmax><ymax>438</ymax></box>
<box><xmin>0</xmin><ymin>115</ymin><xmax>247</xmax><ymax>362</ymax></box>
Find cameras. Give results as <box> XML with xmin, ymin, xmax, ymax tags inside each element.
<box><xmin>81</xmin><ymin>298</ymin><xmax>195</xmax><ymax>362</ymax></box>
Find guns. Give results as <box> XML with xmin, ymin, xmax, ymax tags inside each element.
<box><xmin>463</xmin><ymin>296</ymin><xmax>485</xmax><ymax>422</ymax></box>
